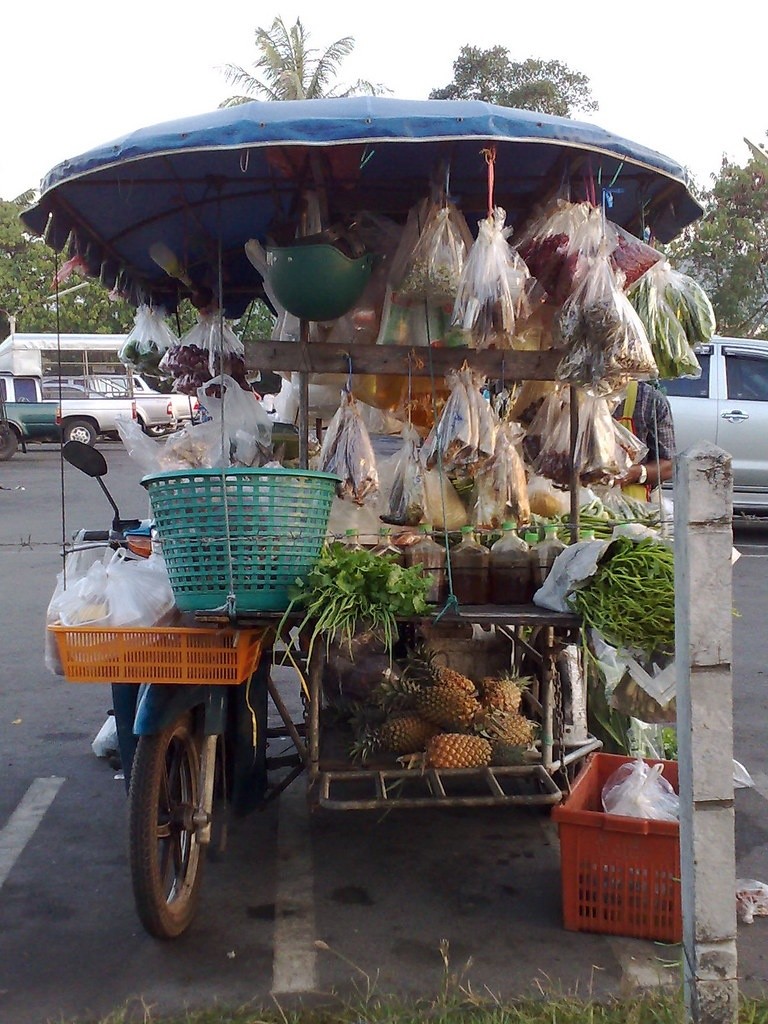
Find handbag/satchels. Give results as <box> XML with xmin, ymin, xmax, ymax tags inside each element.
<box><xmin>44</xmin><ymin>178</ymin><xmax>717</xmax><ymax>675</ymax></box>
<box><xmin>601</xmin><ymin>755</ymin><xmax>680</xmax><ymax>822</ymax></box>
<box><xmin>627</xmin><ymin>716</ymin><xmax>754</xmax><ymax>791</ymax></box>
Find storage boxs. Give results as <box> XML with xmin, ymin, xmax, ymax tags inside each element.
<box><xmin>46</xmin><ymin>468</ymin><xmax>345</xmax><ymax>684</ymax></box>
<box><xmin>552</xmin><ymin>751</ymin><xmax>684</xmax><ymax>942</ymax></box>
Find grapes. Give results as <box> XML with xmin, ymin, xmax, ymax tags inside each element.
<box><xmin>521</xmin><ymin>398</ymin><xmax>584</xmax><ymax>485</ymax></box>
<box><xmin>161</xmin><ymin>344</ymin><xmax>244</xmax><ymax>396</ymax></box>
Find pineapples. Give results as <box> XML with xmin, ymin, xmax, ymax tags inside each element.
<box><xmin>350</xmin><ymin>639</ymin><xmax>541</xmax><ymax>766</ymax></box>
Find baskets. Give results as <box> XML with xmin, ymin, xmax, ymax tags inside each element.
<box><xmin>552</xmin><ymin>752</ymin><xmax>681</xmax><ymax>942</ymax></box>
<box><xmin>47</xmin><ymin>620</ymin><xmax>266</xmax><ymax>685</ymax></box>
<box><xmin>138</xmin><ymin>467</ymin><xmax>343</xmax><ymax>612</ymax></box>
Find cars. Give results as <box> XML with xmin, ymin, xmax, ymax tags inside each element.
<box><xmin>648</xmin><ymin>334</ymin><xmax>768</xmax><ymax>518</ymax></box>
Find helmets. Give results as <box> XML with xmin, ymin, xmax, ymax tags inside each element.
<box><xmin>264</xmin><ymin>243</ymin><xmax>377</xmax><ymax>321</ymax></box>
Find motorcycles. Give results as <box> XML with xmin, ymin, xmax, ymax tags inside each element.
<box><xmin>56</xmin><ymin>437</ymin><xmax>275</xmax><ymax>944</ymax></box>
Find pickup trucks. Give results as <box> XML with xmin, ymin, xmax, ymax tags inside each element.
<box><xmin>0</xmin><ymin>370</ymin><xmax>200</xmax><ymax>463</ymax></box>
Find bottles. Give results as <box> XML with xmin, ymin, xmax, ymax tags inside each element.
<box><xmin>343</xmin><ymin>528</ymin><xmax>368</xmax><ymax>553</ymax></box>
<box><xmin>582</xmin><ymin>531</ymin><xmax>596</xmax><ymax>540</ymax></box>
<box><xmin>530</xmin><ymin>524</ymin><xmax>567</xmax><ymax>595</ymax></box>
<box><xmin>406</xmin><ymin>523</ymin><xmax>446</xmax><ymax>604</ymax></box>
<box><xmin>491</xmin><ymin>522</ymin><xmax>530</xmax><ymax>604</ymax></box>
<box><xmin>450</xmin><ymin>526</ymin><xmax>489</xmax><ymax>605</ymax></box>
<box><xmin>525</xmin><ymin>533</ymin><xmax>538</xmax><ymax>550</ymax></box>
<box><xmin>369</xmin><ymin>528</ymin><xmax>405</xmax><ymax>568</ymax></box>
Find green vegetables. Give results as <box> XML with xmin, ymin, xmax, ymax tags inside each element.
<box><xmin>288</xmin><ymin>544</ymin><xmax>434</xmax><ymax>643</ymax></box>
<box><xmin>573</xmin><ymin>536</ymin><xmax>675</xmax><ymax>657</ymax></box>
<box><xmin>560</xmin><ymin>504</ymin><xmax>658</xmax><ymax>544</ymax></box>
